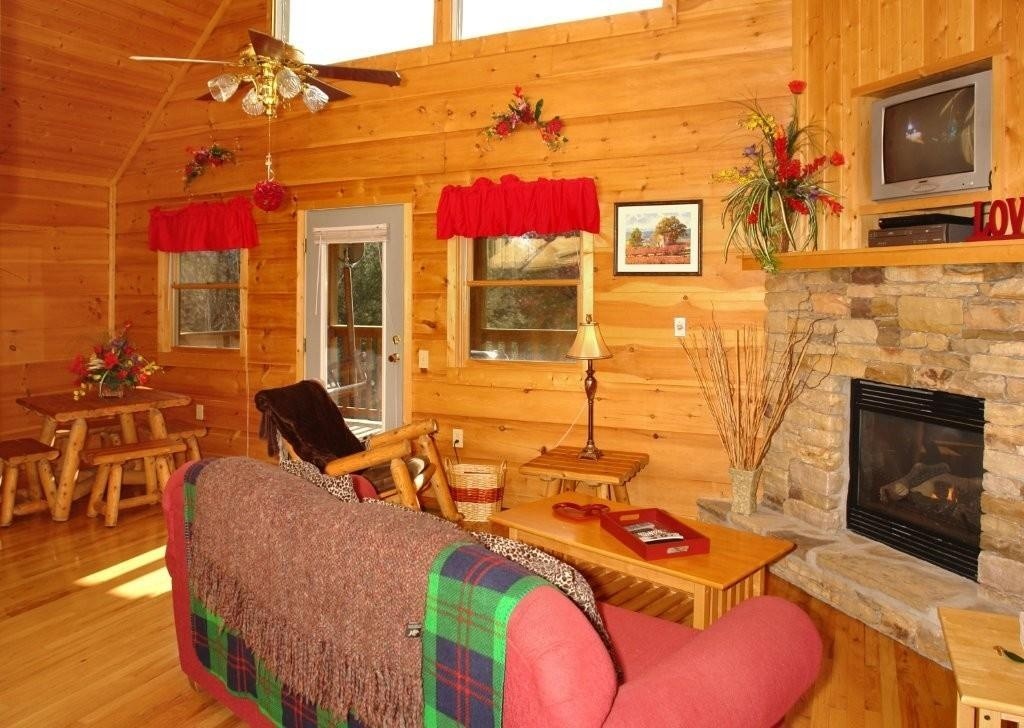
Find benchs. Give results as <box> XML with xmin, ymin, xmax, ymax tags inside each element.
<box><xmin>0</xmin><ymin>417</ymin><xmax>209</xmax><ymax>527</ymax></box>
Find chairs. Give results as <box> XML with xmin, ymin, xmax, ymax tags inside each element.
<box><xmin>254</xmin><ymin>380</ymin><xmax>468</xmax><ymax>523</ymax></box>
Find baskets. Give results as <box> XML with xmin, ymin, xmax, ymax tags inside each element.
<box><xmin>443</xmin><ymin>456</ymin><xmax>508</xmax><ymax>522</ymax></box>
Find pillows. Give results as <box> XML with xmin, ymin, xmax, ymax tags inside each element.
<box><xmin>361</xmin><ymin>497</ymin><xmax>460</xmax><ymax>531</ymax></box>
<box><xmin>470</xmin><ymin>531</ymin><xmax>620</xmax><ymax>675</ymax></box>
<box><xmin>273</xmin><ymin>457</ymin><xmax>359</xmax><ymax>504</ymax></box>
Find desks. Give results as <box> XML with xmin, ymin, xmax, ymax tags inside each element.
<box><xmin>14</xmin><ymin>387</ymin><xmax>190</xmax><ymax>520</ymax></box>
<box><xmin>938</xmin><ymin>606</ymin><xmax>1024</xmax><ymax>728</ymax></box>
<box><xmin>520</xmin><ymin>448</ymin><xmax>650</xmax><ymax>503</ymax></box>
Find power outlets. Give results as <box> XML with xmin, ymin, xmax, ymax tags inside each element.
<box><xmin>195</xmin><ymin>404</ymin><xmax>204</xmax><ymax>422</ymax></box>
<box><xmin>419</xmin><ymin>351</ymin><xmax>428</xmax><ymax>368</ymax></box>
<box><xmin>674</xmin><ymin>317</ymin><xmax>686</xmax><ymax>337</ymax></box>
<box><xmin>453</xmin><ymin>429</ymin><xmax>464</xmax><ymax>448</ymax></box>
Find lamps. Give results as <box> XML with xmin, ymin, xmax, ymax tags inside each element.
<box><xmin>209</xmin><ymin>56</ymin><xmax>328</xmax><ymax>180</ymax></box>
<box><xmin>567</xmin><ymin>315</ymin><xmax>610</xmax><ymax>459</ymax></box>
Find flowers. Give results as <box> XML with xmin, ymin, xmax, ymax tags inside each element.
<box><xmin>716</xmin><ymin>78</ymin><xmax>848</xmax><ymax>272</ymax></box>
<box><xmin>72</xmin><ymin>329</ymin><xmax>163</xmax><ymax>397</ymax></box>
<box><xmin>183</xmin><ymin>134</ymin><xmax>239</xmax><ymax>188</ymax></box>
<box><xmin>474</xmin><ymin>84</ymin><xmax>569</xmax><ymax>163</ymax></box>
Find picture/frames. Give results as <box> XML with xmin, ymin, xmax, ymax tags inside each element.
<box><xmin>612</xmin><ymin>198</ymin><xmax>703</xmax><ymax>276</ymax></box>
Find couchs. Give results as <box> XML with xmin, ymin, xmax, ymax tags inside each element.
<box><xmin>166</xmin><ymin>455</ymin><xmax>823</xmax><ymax>728</ymax></box>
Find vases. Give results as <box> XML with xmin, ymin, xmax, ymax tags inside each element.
<box><xmin>770</xmin><ymin>197</ymin><xmax>795</xmax><ymax>252</ymax></box>
<box><xmin>727</xmin><ymin>463</ymin><xmax>761</xmax><ymax>514</ymax></box>
<box><xmin>100</xmin><ymin>381</ymin><xmax>125</xmax><ymax>397</ymax></box>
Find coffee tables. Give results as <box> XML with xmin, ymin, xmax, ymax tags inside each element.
<box><xmin>487</xmin><ymin>492</ymin><xmax>794</xmax><ymax>629</ymax></box>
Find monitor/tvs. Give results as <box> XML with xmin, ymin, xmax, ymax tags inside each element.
<box><xmin>871</xmin><ymin>69</ymin><xmax>993</xmax><ymax>205</ymax></box>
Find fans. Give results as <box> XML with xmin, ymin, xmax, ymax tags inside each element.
<box><xmin>129</xmin><ymin>0</ymin><xmax>400</xmax><ymax>104</ymax></box>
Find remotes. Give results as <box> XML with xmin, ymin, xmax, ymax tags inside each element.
<box><xmin>624</xmin><ymin>521</ymin><xmax>684</xmax><ymax>542</ymax></box>
